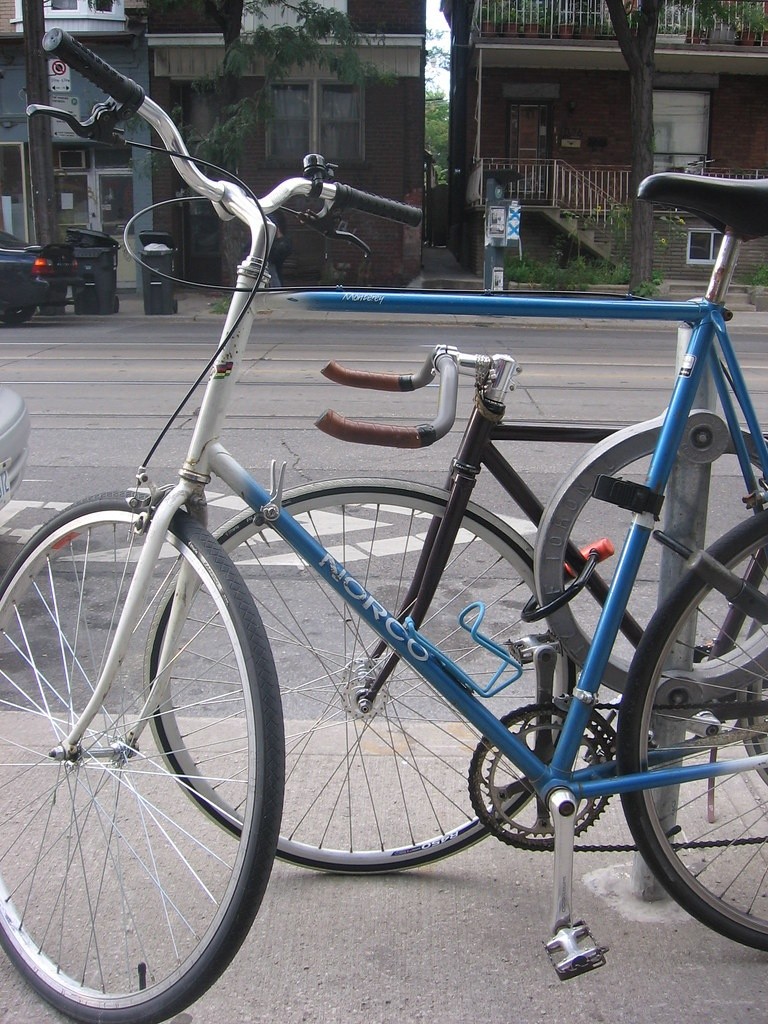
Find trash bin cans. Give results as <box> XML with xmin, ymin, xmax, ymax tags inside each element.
<box><xmin>65</xmin><ymin>227</ymin><xmax>120</xmax><ymax>315</ymax></box>
<box><xmin>138</xmin><ymin>230</ymin><xmax>178</xmax><ymax>315</ymax></box>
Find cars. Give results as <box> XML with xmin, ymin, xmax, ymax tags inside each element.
<box><xmin>0</xmin><ymin>229</ymin><xmax>79</xmax><ymax>324</ymax></box>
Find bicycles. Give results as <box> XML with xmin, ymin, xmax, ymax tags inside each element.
<box><xmin>0</xmin><ymin>25</ymin><xmax>768</xmax><ymax>1024</ymax></box>
